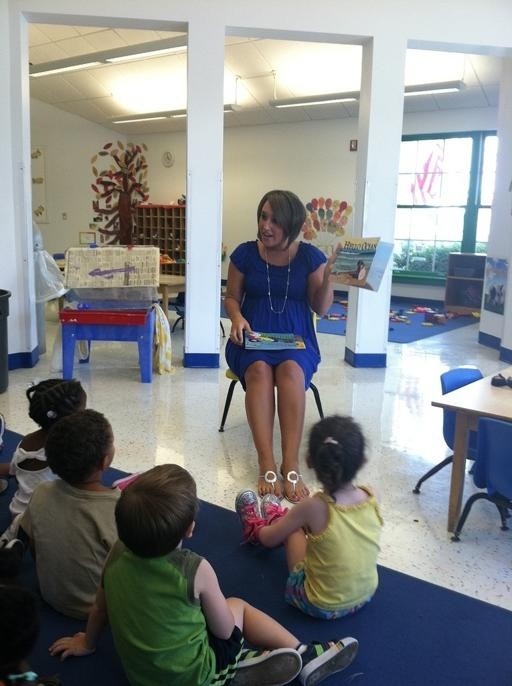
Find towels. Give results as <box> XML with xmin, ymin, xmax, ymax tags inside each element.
<box><xmin>149</xmin><ymin>303</ymin><xmax>173</xmax><ymax>376</ymax></box>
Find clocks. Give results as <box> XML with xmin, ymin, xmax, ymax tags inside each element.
<box><xmin>161</xmin><ymin>150</ymin><xmax>175</xmax><ymax>166</ymax></box>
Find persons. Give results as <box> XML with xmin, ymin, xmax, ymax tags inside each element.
<box><xmin>222</xmin><ymin>187</ymin><xmax>344</xmax><ymax>507</ymax></box>
<box><xmin>15</xmin><ymin>408</ymin><xmax>125</xmax><ymax>623</ymax></box>
<box><xmin>46</xmin><ymin>461</ymin><xmax>360</xmax><ymax>685</ymax></box>
<box><xmin>0</xmin><ymin>412</ymin><xmax>12</xmax><ymax>495</ymax></box>
<box><xmin>0</xmin><ymin>588</ymin><xmax>70</xmax><ymax>685</ymax></box>
<box><xmin>0</xmin><ymin>546</ymin><xmax>27</xmax><ymax>591</ymax></box>
<box><xmin>0</xmin><ymin>377</ymin><xmax>89</xmax><ymax>562</ymax></box>
<box><xmin>234</xmin><ymin>413</ymin><xmax>384</xmax><ymax>621</ymax></box>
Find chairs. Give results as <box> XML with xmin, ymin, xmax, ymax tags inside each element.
<box><xmin>175</xmin><ymin>288</ymin><xmax>227</xmax><ymax>339</ymax></box>
<box><xmin>447</xmin><ymin>417</ymin><xmax>512</xmax><ymax>543</ymax></box>
<box><xmin>408</xmin><ymin>365</ymin><xmax>478</xmax><ymax>497</ymax></box>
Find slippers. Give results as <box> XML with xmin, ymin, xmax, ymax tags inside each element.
<box><xmin>280</xmin><ymin>466</ymin><xmax>310</xmax><ymax>504</ymax></box>
<box><xmin>257</xmin><ymin>469</ymin><xmax>283</xmax><ymax>500</ymax></box>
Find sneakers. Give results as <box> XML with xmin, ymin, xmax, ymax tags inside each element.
<box><xmin>300</xmin><ymin>637</ymin><xmax>359</xmax><ymax>685</ymax></box>
<box><xmin>260</xmin><ymin>493</ymin><xmax>288</xmax><ymax>526</ymax></box>
<box><xmin>237</xmin><ymin>489</ymin><xmax>262</xmax><ymax>545</ymax></box>
<box><xmin>233</xmin><ymin>648</ymin><xmax>302</xmax><ymax>686</ymax></box>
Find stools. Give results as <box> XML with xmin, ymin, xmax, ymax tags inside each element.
<box><xmin>218</xmin><ymin>311</ymin><xmax>323</xmax><ymax>433</ymax></box>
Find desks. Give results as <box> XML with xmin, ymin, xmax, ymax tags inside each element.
<box><xmin>431</xmin><ymin>365</ymin><xmax>512</xmax><ymax>535</ymax></box>
<box><xmin>64</xmin><ymin>301</ymin><xmax>164</xmax><ymax>384</ymax></box>
<box><xmin>58</xmin><ymin>272</ymin><xmax>185</xmax><ymax>325</ymax></box>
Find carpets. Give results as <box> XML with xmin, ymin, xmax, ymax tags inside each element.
<box><xmin>0</xmin><ymin>429</ymin><xmax>512</xmax><ymax>686</ymax></box>
<box><xmin>158</xmin><ymin>287</ymin><xmax>481</xmax><ymax>345</ymax></box>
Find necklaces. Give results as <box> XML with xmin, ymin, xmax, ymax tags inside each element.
<box><xmin>264</xmin><ymin>246</ymin><xmax>291</xmax><ymax>314</ymax></box>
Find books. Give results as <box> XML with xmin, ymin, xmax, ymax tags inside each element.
<box><xmin>292</xmin><ymin>334</ymin><xmax>306</xmax><ymax>349</ymax></box>
<box><xmin>243</xmin><ymin>330</ymin><xmax>297</xmax><ymax>350</ymax></box>
<box><xmin>326</xmin><ymin>236</ymin><xmax>395</xmax><ymax>292</ymax></box>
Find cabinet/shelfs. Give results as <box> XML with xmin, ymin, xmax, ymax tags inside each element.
<box><xmin>130</xmin><ymin>204</ymin><xmax>186</xmax><ymax>276</ymax></box>
<box><xmin>443</xmin><ymin>251</ymin><xmax>487</xmax><ymax>315</ymax></box>
